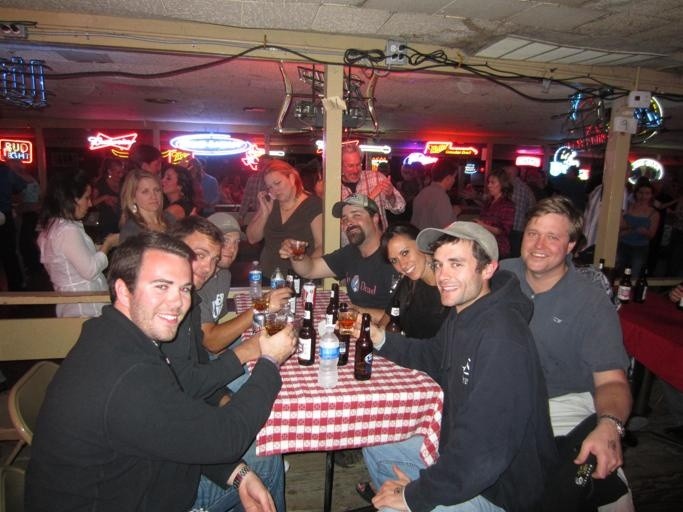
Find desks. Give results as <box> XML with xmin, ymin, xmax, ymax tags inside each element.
<box><xmin>234</xmin><ymin>286</ymin><xmax>446</xmax><ymax>512</ymax></box>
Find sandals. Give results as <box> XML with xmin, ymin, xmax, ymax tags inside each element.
<box><xmin>358</xmin><ymin>481</ymin><xmax>376</xmax><ymax>503</ymax></box>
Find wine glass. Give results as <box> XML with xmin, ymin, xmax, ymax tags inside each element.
<box><xmin>290</xmin><ymin>240</ymin><xmax>307</xmax><ymax>261</ymax></box>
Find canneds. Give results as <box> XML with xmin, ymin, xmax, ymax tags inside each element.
<box><xmin>253</xmin><ymin>313</ymin><xmax>266</xmax><ymax>335</ymax></box>
<box><xmin>302</xmin><ymin>282</ymin><xmax>316</xmax><ymax>309</ymax></box>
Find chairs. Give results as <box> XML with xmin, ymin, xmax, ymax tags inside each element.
<box><xmin>0</xmin><ymin>463</ymin><xmax>26</xmax><ymax>511</ymax></box>
<box><xmin>0</xmin><ymin>362</ymin><xmax>60</xmax><ymax>465</ymax></box>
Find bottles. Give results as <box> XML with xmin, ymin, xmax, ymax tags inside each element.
<box><xmin>247</xmin><ymin>260</ymin><xmax>405</xmax><ymax>388</ymax></box>
<box><xmin>598</xmin><ymin>258</ymin><xmax>650</xmax><ymax>305</ymax></box>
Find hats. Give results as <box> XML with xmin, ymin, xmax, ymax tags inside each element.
<box><xmin>415</xmin><ymin>221</ymin><xmax>499</xmax><ymax>261</ymax></box>
<box><xmin>333</xmin><ymin>195</ymin><xmax>379</xmax><ymax>218</ymax></box>
<box><xmin>206</xmin><ymin>213</ymin><xmax>242</xmax><ymax>238</ymax></box>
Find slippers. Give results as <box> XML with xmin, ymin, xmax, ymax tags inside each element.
<box><xmin>335</xmin><ymin>448</ymin><xmax>364</xmax><ymax>465</ymax></box>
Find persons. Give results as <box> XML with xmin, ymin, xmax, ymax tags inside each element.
<box><xmin>279</xmin><ymin>142</ymin><xmax>676</xmax><ymax>338</ymax></box>
<box><xmin>25</xmin><ymin>231</ymin><xmax>297</xmax><ymax>511</ymax></box>
<box><xmin>339</xmin><ymin>221</ymin><xmax>561</xmax><ymax>511</ymax></box>
<box><xmin>498</xmin><ymin>193</ymin><xmax>635</xmax><ymax>512</ymax></box>
<box><xmin>1</xmin><ymin>142</ymin><xmax>324</xmax><ymax>406</ymax></box>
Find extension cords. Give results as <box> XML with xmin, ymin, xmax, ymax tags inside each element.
<box><xmin>0</xmin><ymin>23</ymin><xmax>29</xmax><ymax>39</ymax></box>
<box><xmin>383</xmin><ymin>41</ymin><xmax>408</xmax><ymax>65</ymax></box>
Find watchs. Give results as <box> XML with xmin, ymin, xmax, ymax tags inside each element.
<box><xmin>596</xmin><ymin>414</ymin><xmax>627</xmax><ymax>438</ymax></box>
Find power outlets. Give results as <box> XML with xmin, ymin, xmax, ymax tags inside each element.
<box><xmin>387</xmin><ymin>39</ymin><xmax>408</xmax><ymax>65</ymax></box>
<box><xmin>0</xmin><ymin>24</ymin><xmax>26</xmax><ymax>38</ymax></box>
